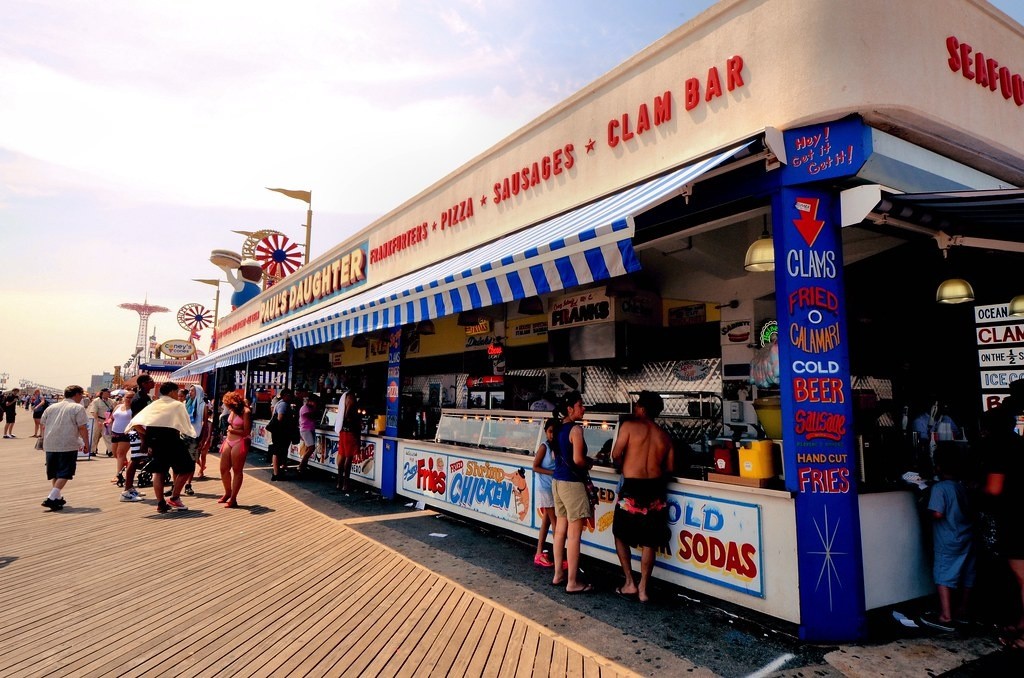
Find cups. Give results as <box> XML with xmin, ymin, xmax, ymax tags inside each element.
<box><xmin>937</xmin><ymin>422</ymin><xmax>952</xmax><ymax>440</ymax></box>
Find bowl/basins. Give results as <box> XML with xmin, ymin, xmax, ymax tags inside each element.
<box><xmin>752</xmin><ymin>396</ymin><xmax>783</xmax><ymax>440</ymax></box>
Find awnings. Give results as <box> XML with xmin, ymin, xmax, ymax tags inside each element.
<box><xmin>170</xmin><ymin>138</ymin><xmax>756</xmax><ymax>378</ymax></box>
<box><xmin>877</xmin><ymin>188</ymin><xmax>1024</xmax><ymax>252</ymax></box>
<box><xmin>149</xmin><ymin>371</ymin><xmax>201</xmax><ymax>386</ymax></box>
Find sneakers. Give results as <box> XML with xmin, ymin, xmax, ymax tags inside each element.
<box><xmin>157</xmin><ymin>501</ymin><xmax>172</xmax><ymax>512</ymax></box>
<box><xmin>129</xmin><ymin>488</ymin><xmax>146</xmax><ymax>496</ymax></box>
<box><xmin>167</xmin><ymin>496</ymin><xmax>188</xmax><ymax>510</ymax></box>
<box><xmin>120</xmin><ymin>491</ymin><xmax>143</xmax><ymax>502</ymax></box>
<box><xmin>224</xmin><ymin>498</ymin><xmax>237</xmax><ymax>508</ymax></box>
<box><xmin>58</xmin><ymin>497</ymin><xmax>66</xmax><ymax>504</ymax></box>
<box><xmin>41</xmin><ymin>498</ymin><xmax>63</xmax><ymax>510</ymax></box>
<box><xmin>218</xmin><ymin>493</ymin><xmax>231</xmax><ymax>503</ymax></box>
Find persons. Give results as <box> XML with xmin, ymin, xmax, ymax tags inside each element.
<box><xmin>533</xmin><ymin>420</ymin><xmax>568</xmax><ymax>570</ymax></box>
<box><xmin>915</xmin><ymin>382</ymin><xmax>1024</xmax><ymax>649</ymax></box>
<box><xmin>530</xmin><ymin>390</ymin><xmax>556</xmax><ymax>410</ymax></box>
<box><xmin>84</xmin><ymin>389</ymin><xmax>156</xmax><ymax>481</ymax></box>
<box><xmin>40</xmin><ymin>385</ymin><xmax>88</xmax><ymax>511</ymax></box>
<box><xmin>552</xmin><ymin>392</ymin><xmax>599</xmax><ymax>594</ymax></box>
<box><xmin>612</xmin><ymin>391</ymin><xmax>673</xmax><ymax>607</ymax></box>
<box><xmin>119</xmin><ymin>374</ymin><xmax>155</xmax><ymax>502</ymax></box>
<box><xmin>124</xmin><ymin>382</ymin><xmax>198</xmax><ymax>513</ymax></box>
<box><xmin>271</xmin><ymin>386</ymin><xmax>315</xmax><ymax>481</ymax></box>
<box><xmin>218</xmin><ymin>392</ymin><xmax>252</xmax><ymax>507</ymax></box>
<box><xmin>164</xmin><ymin>385</ymin><xmax>232</xmax><ymax>496</ymax></box>
<box><xmin>0</xmin><ymin>388</ymin><xmax>66</xmax><ymax>438</ymax></box>
<box><xmin>335</xmin><ymin>378</ymin><xmax>361</xmax><ymax>492</ymax></box>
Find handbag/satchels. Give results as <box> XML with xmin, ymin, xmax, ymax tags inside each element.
<box><xmin>38</xmin><ymin>396</ymin><xmax>50</xmax><ymax>410</ymax></box>
<box><xmin>579</xmin><ymin>474</ymin><xmax>598</xmax><ymax>508</ymax></box>
<box><xmin>265</xmin><ymin>412</ymin><xmax>287</xmax><ymax>439</ymax></box>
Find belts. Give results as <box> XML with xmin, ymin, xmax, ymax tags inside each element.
<box><xmin>98</xmin><ymin>416</ymin><xmax>106</xmax><ymax>420</ymax></box>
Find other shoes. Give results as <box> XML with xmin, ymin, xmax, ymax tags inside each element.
<box><xmin>920</xmin><ymin>613</ymin><xmax>955</xmax><ymax>632</ymax></box>
<box><xmin>164</xmin><ymin>485</ymin><xmax>175</xmax><ymax>496</ymax></box>
<box><xmin>271</xmin><ymin>466</ymin><xmax>349</xmax><ymax>493</ymax></box>
<box><xmin>3</xmin><ymin>434</ymin><xmax>126</xmax><ymax>482</ymax></box>
<box><xmin>185</xmin><ymin>484</ymin><xmax>194</xmax><ymax>495</ymax></box>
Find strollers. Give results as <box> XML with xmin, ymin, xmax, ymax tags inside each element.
<box><xmin>116</xmin><ymin>454</ymin><xmax>172</xmax><ymax>488</ymax></box>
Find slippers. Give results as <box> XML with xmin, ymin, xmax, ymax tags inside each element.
<box><xmin>551</xmin><ymin>577</ymin><xmax>569</xmax><ymax>587</ymax></box>
<box><xmin>533</xmin><ymin>553</ymin><xmax>554</xmax><ymax>567</ymax></box>
<box><xmin>613</xmin><ymin>586</ymin><xmax>635</xmax><ymax>602</ymax></box>
<box><xmin>562</xmin><ymin>560</ymin><xmax>568</xmax><ymax>571</ymax></box>
<box><xmin>565</xmin><ymin>581</ymin><xmax>600</xmax><ymax>594</ymax></box>
<box><xmin>634</xmin><ymin>589</ymin><xmax>648</xmax><ymax>604</ymax></box>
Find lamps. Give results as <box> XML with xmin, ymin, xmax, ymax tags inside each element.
<box><xmin>329</xmin><ymin>339</ymin><xmax>344</xmax><ymax>352</ymax></box>
<box><xmin>605</xmin><ymin>278</ymin><xmax>627</xmax><ymax>296</ymax></box>
<box><xmin>351</xmin><ymin>334</ymin><xmax>368</xmax><ymax>348</ymax></box>
<box><xmin>517</xmin><ymin>295</ymin><xmax>544</xmax><ymax>315</ymax></box>
<box><xmin>743</xmin><ymin>214</ymin><xmax>775</xmax><ymax>271</ymax></box>
<box><xmin>936</xmin><ymin>278</ymin><xmax>974</xmax><ymax>303</ymax></box>
<box><xmin>415</xmin><ymin>319</ymin><xmax>435</xmax><ymax>335</ymax></box>
<box><xmin>1009</xmin><ymin>294</ymin><xmax>1024</xmax><ymax>317</ymax></box>
<box><xmin>457</xmin><ymin>310</ymin><xmax>479</xmax><ymax>326</ymax></box>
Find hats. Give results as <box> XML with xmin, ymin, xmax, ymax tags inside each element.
<box><xmin>101</xmin><ymin>387</ymin><xmax>109</xmax><ymax>392</ymax></box>
